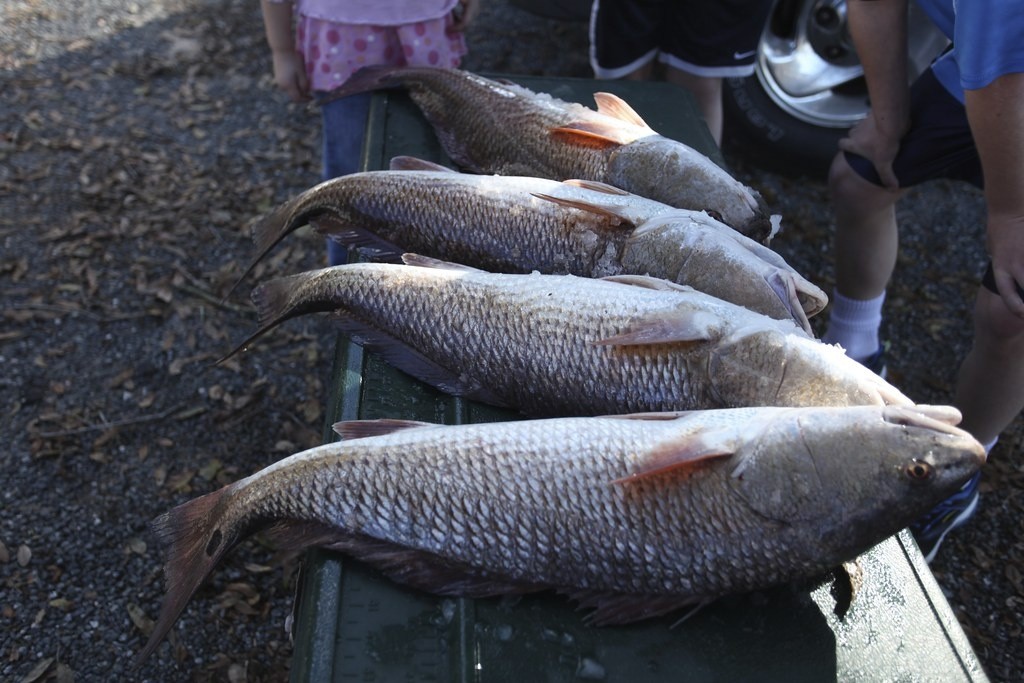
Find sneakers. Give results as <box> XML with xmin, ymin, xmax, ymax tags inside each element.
<box><xmin>859</xmin><ymin>345</ymin><xmax>890</xmax><ymax>379</ymax></box>
<box><xmin>906</xmin><ymin>472</ymin><xmax>988</xmax><ymax>565</ymax></box>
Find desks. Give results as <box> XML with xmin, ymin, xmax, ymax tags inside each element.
<box><xmin>288</xmin><ymin>68</ymin><xmax>992</xmax><ymax>683</ymax></box>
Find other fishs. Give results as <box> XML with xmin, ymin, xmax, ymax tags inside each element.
<box><xmin>122</xmin><ymin>62</ymin><xmax>986</xmax><ymax>671</ymax></box>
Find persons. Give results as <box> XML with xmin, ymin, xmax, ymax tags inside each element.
<box><xmin>589</xmin><ymin>0</ymin><xmax>773</xmax><ymax>149</ymax></box>
<box><xmin>261</xmin><ymin>1</ymin><xmax>478</xmax><ymax>269</ymax></box>
<box><xmin>822</xmin><ymin>0</ymin><xmax>1024</xmax><ymax>563</ymax></box>
